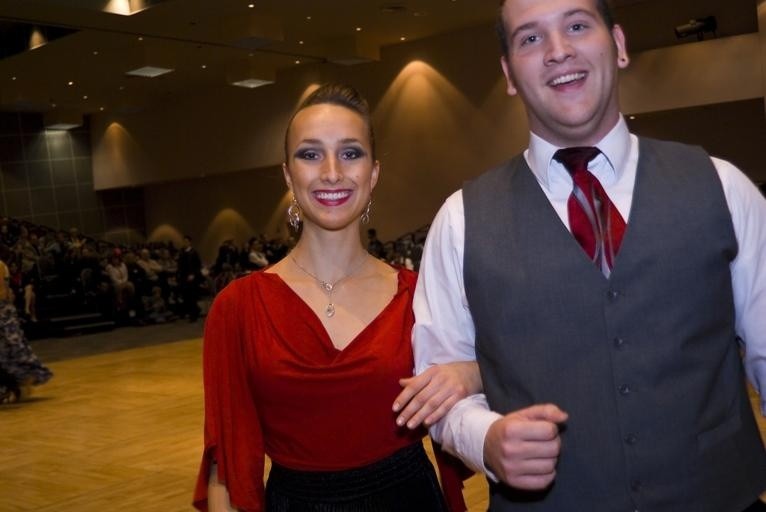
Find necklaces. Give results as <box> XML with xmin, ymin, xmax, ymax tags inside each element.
<box><xmin>287</xmin><ymin>250</ymin><xmax>372</xmax><ymax>319</ymax></box>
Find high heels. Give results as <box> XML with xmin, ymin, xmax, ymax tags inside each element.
<box><xmin>0</xmin><ymin>391</ymin><xmax>11</xmax><ymax>403</ymax></box>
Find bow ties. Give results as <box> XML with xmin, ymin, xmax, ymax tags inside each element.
<box><xmin>553</xmin><ymin>146</ymin><xmax>626</xmax><ymax>273</ymax></box>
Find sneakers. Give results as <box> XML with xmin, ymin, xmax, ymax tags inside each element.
<box><xmin>24</xmin><ymin>307</ymin><xmax>38</xmax><ymax>322</ymax></box>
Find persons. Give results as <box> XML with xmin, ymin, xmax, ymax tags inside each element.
<box><xmin>412</xmin><ymin>0</ymin><xmax>766</xmax><ymax>510</ymax></box>
<box><xmin>1</xmin><ymin>216</ymin><xmax>304</xmax><ymax>403</ymax></box>
<box><xmin>189</xmin><ymin>81</ymin><xmax>486</xmax><ymax>511</ymax></box>
<box><xmin>361</xmin><ymin>225</ymin><xmax>429</xmax><ymax>273</ymax></box>
